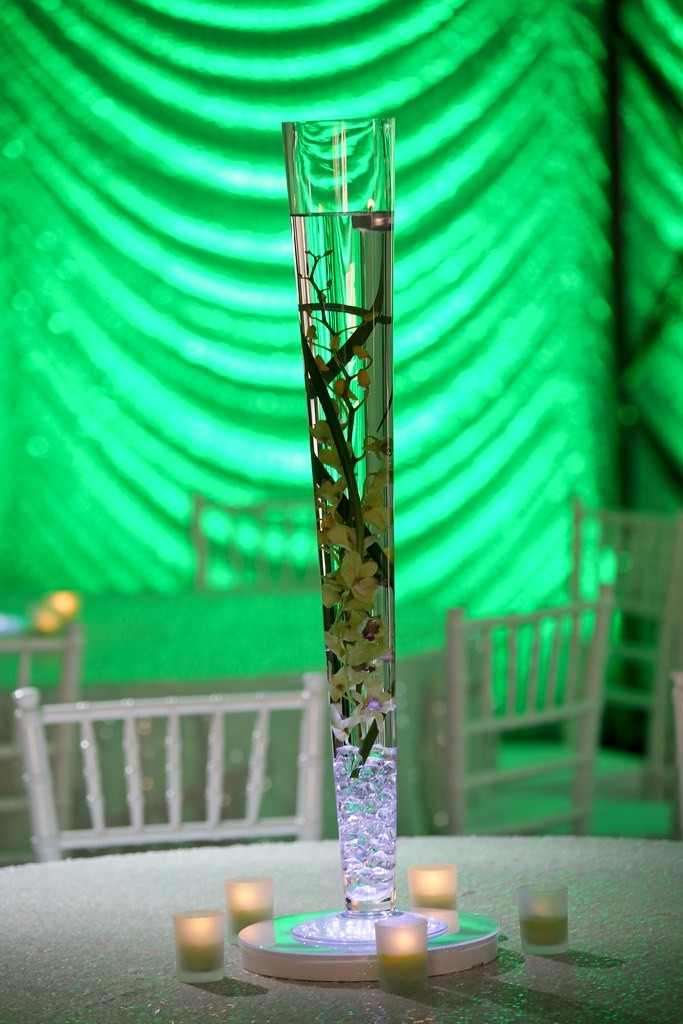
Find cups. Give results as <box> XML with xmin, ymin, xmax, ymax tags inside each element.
<box><xmin>375</xmin><ymin>917</ymin><xmax>429</xmax><ymax>996</ymax></box>
<box><xmin>516</xmin><ymin>882</ymin><xmax>569</xmax><ymax>956</ymax></box>
<box><xmin>224</xmin><ymin>876</ymin><xmax>275</xmax><ymax>947</ymax></box>
<box><xmin>172</xmin><ymin>909</ymin><xmax>225</xmax><ymax>984</ymax></box>
<box><xmin>408</xmin><ymin>863</ymin><xmax>459</xmax><ymax>911</ymax></box>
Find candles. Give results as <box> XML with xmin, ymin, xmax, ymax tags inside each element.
<box><xmin>223</xmin><ymin>877</ymin><xmax>277</xmax><ymax>944</ymax></box>
<box><xmin>171</xmin><ymin>907</ymin><xmax>224</xmax><ymax>984</ymax></box>
<box><xmin>374</xmin><ymin>917</ymin><xmax>429</xmax><ymax>995</ymax></box>
<box><xmin>515</xmin><ymin>883</ymin><xmax>569</xmax><ymax>955</ymax></box>
<box><xmin>405</xmin><ymin>863</ymin><xmax>461</xmax><ymax>933</ymax></box>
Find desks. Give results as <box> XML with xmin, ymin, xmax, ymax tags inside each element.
<box><xmin>0</xmin><ymin>835</ymin><xmax>683</xmax><ymax>1024</ymax></box>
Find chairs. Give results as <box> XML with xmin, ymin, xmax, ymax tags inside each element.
<box><xmin>0</xmin><ymin>491</ymin><xmax>683</xmax><ymax>864</ymax></box>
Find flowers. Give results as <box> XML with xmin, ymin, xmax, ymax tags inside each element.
<box><xmin>281</xmin><ymin>108</ymin><xmax>396</xmax><ymax>774</ymax></box>
